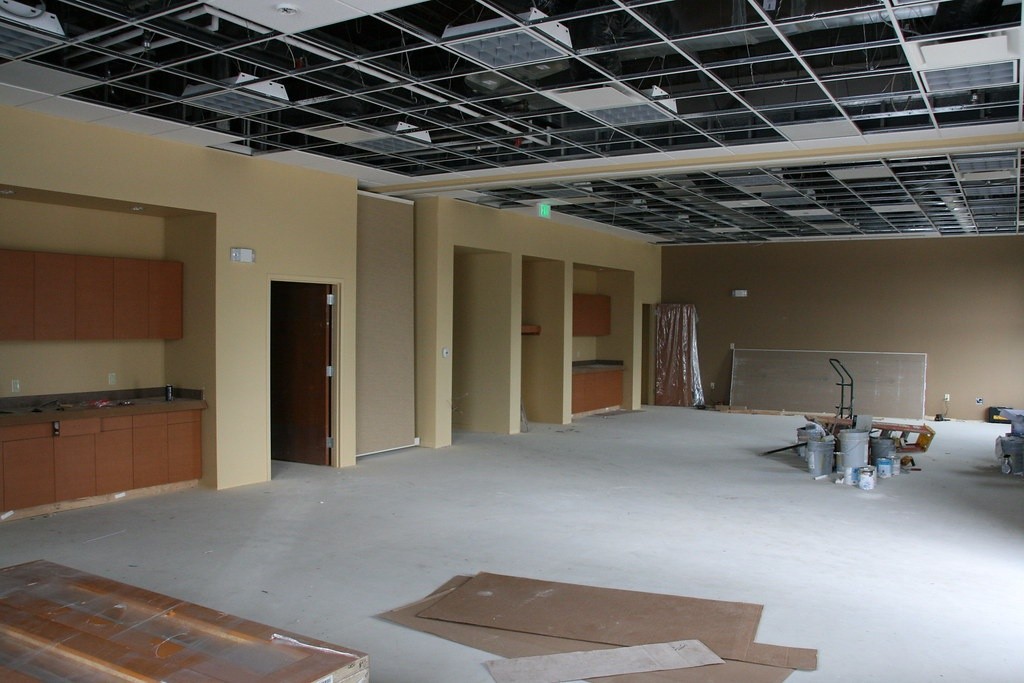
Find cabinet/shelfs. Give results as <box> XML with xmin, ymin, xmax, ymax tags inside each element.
<box><xmin>33</xmin><ymin>252</ymin><xmax>113</xmax><ymax>340</ymax></box>
<box><xmin>54</xmin><ymin>416</ymin><xmax>134</xmax><ymax>513</ymax></box>
<box><xmin>573</xmin><ymin>294</ymin><xmax>611</xmax><ymax>337</ymax></box>
<box><xmin>0</xmin><ymin>249</ymin><xmax>34</xmax><ymax>341</ymax></box>
<box><xmin>132</xmin><ymin>409</ymin><xmax>203</xmax><ymax>499</ymax></box>
<box><xmin>0</xmin><ymin>422</ymin><xmax>54</xmax><ymax>523</ymax></box>
<box><xmin>114</xmin><ymin>257</ymin><xmax>183</xmax><ymax>340</ymax></box>
<box><xmin>572</xmin><ymin>371</ymin><xmax>623</xmax><ymax>419</ymax></box>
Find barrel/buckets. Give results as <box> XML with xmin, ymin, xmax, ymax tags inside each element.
<box><xmin>796</xmin><ymin>425</ymin><xmax>901</xmax><ymax>491</ymax></box>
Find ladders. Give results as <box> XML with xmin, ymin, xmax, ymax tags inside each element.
<box><xmin>805</xmin><ymin>414</ymin><xmax>936</xmax><ymax>452</ymax></box>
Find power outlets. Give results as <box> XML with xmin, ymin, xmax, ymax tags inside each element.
<box><xmin>944</xmin><ymin>393</ymin><xmax>950</xmax><ymax>402</ymax></box>
<box><xmin>108</xmin><ymin>373</ymin><xmax>117</xmax><ymax>385</ymax></box>
<box><xmin>11</xmin><ymin>379</ymin><xmax>21</xmax><ymax>393</ymax></box>
<box><xmin>710</xmin><ymin>382</ymin><xmax>715</xmax><ymax>390</ymax></box>
